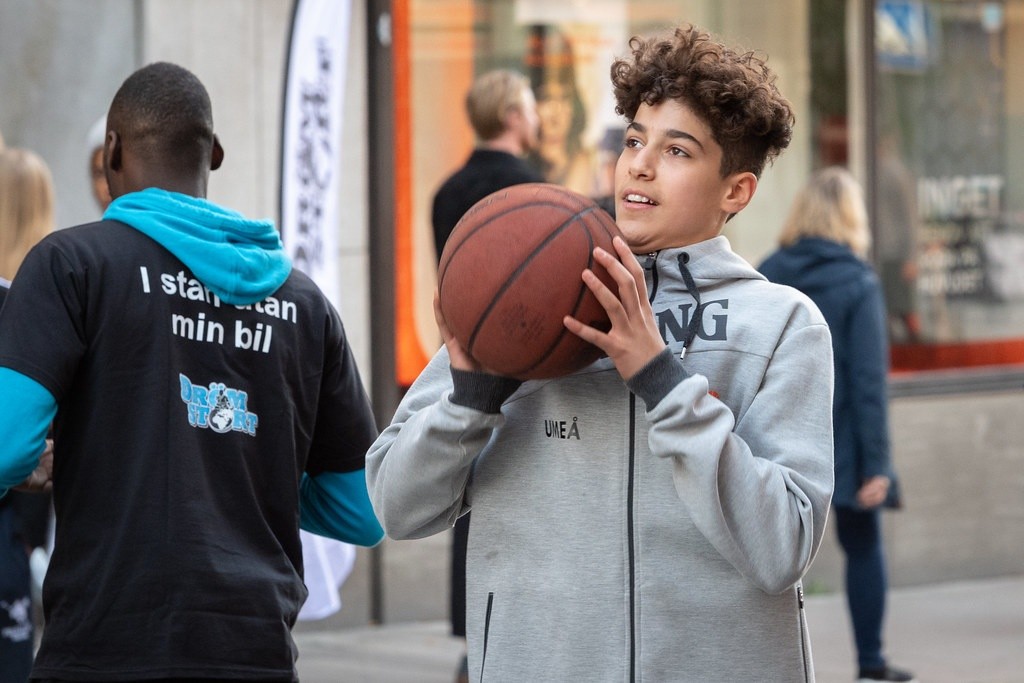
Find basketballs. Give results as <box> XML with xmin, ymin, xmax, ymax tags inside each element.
<box><xmin>435</xmin><ymin>179</ymin><xmax>631</xmax><ymax>384</ymax></box>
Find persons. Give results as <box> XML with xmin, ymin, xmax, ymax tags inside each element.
<box><xmin>365</xmin><ymin>25</ymin><xmax>834</xmax><ymax>683</ymax></box>
<box><xmin>757</xmin><ymin>167</ymin><xmax>917</xmax><ymax>683</ymax></box>
<box><xmin>0</xmin><ymin>61</ymin><xmax>386</xmax><ymax>683</ymax></box>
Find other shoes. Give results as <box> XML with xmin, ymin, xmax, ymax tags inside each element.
<box><xmin>859</xmin><ymin>663</ymin><xmax>913</xmax><ymax>682</ymax></box>
